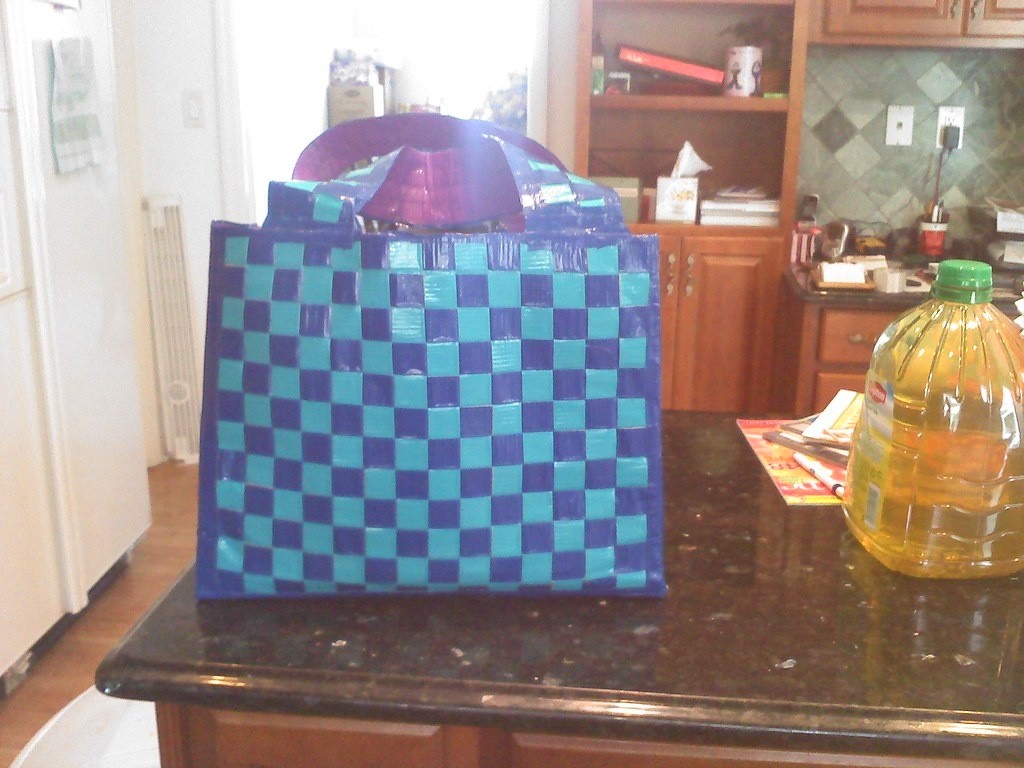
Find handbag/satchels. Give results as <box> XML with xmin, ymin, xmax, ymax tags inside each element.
<box><xmin>194</xmin><ymin>114</ymin><xmax>667</xmax><ymax>599</ymax></box>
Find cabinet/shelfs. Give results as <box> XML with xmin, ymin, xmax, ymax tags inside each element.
<box><xmin>780</xmin><ymin>253</ymin><xmax>1024</xmax><ymax>420</ymax></box>
<box><xmin>808</xmin><ymin>0</ymin><xmax>1024</xmax><ymax>49</ymax></box>
<box><xmin>571</xmin><ymin>1</ymin><xmax>812</xmax><ymax>411</ymax></box>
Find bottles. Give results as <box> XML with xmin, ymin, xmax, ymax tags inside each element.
<box><xmin>842</xmin><ymin>258</ymin><xmax>1024</xmax><ymax>576</ymax></box>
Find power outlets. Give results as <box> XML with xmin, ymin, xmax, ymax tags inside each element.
<box><xmin>934</xmin><ymin>104</ymin><xmax>965</xmax><ymax>149</ymax></box>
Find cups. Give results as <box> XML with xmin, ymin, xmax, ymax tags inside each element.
<box><xmin>723</xmin><ymin>45</ymin><xmax>762</xmax><ymax>96</ymax></box>
<box><xmin>920</xmin><ymin>222</ymin><xmax>948</xmax><ymax>256</ymax></box>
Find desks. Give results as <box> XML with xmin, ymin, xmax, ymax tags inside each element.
<box><xmin>97</xmin><ymin>410</ymin><xmax>1024</xmax><ymax>768</ymax></box>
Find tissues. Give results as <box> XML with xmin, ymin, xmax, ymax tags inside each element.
<box><xmin>654</xmin><ymin>140</ymin><xmax>713</xmax><ymax>224</ymax></box>
